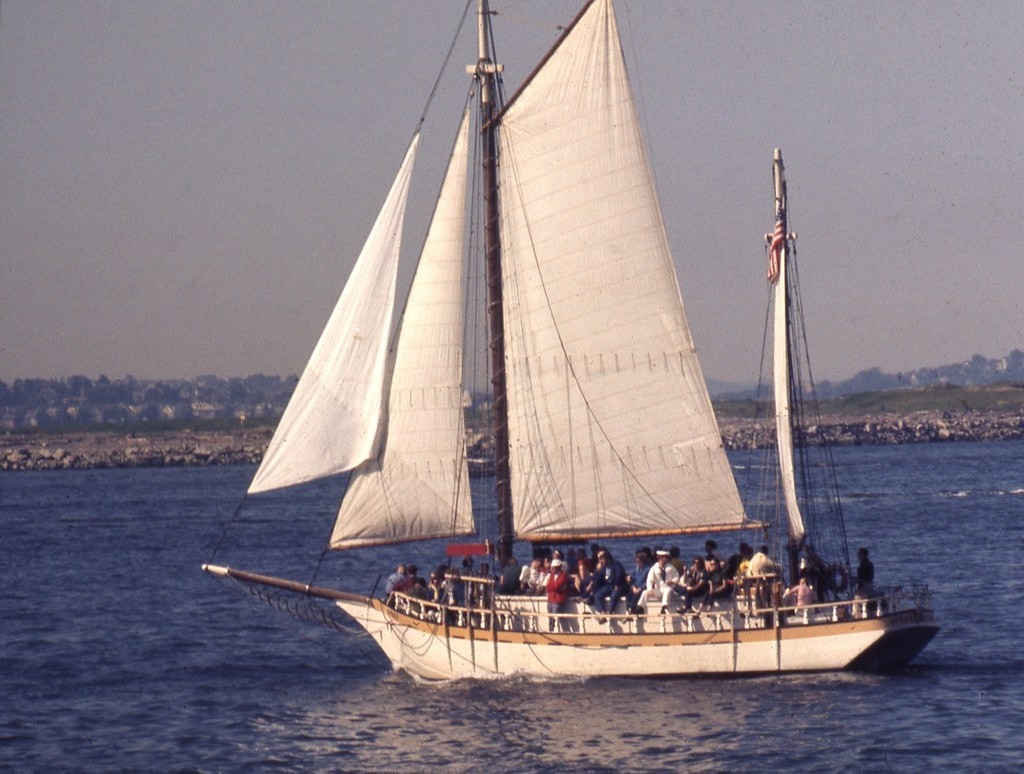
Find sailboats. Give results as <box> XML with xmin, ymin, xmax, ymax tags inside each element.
<box><xmin>201</xmin><ymin>2</ymin><xmax>941</xmax><ymax>681</ymax></box>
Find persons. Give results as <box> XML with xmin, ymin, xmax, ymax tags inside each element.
<box><xmin>385</xmin><ymin>539</ymin><xmax>874</xmax><ymax>622</ymax></box>
